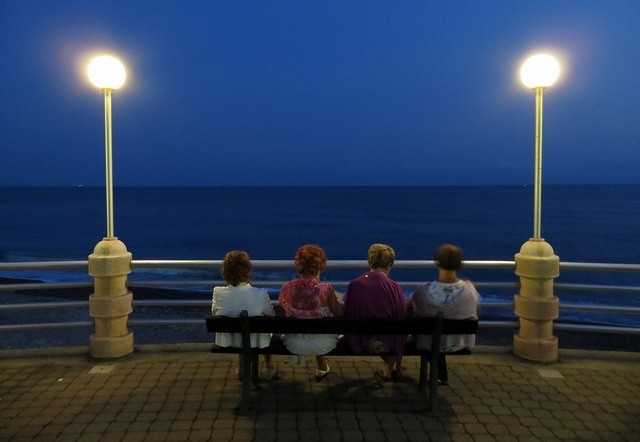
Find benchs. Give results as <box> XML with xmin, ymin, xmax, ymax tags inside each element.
<box><xmin>205</xmin><ymin>310</ymin><xmax>479</xmax><ymax>419</ymax></box>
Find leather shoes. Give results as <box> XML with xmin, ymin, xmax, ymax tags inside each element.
<box><xmin>376</xmin><ymin>367</ymin><xmax>392</xmax><ymax>381</ymax></box>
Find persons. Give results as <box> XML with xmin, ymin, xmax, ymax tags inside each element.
<box><xmin>211</xmin><ymin>251</ymin><xmax>276</xmax><ymax>369</ymax></box>
<box><xmin>413</xmin><ymin>244</ymin><xmax>481</xmax><ymax>385</ymax></box>
<box><xmin>278</xmin><ymin>243</ymin><xmax>341</xmax><ymax>382</ymax></box>
<box><xmin>342</xmin><ymin>243</ymin><xmax>407</xmax><ymax>379</ymax></box>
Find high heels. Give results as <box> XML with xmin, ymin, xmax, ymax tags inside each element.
<box><xmin>314</xmin><ymin>363</ymin><xmax>330</xmax><ymax>383</ymax></box>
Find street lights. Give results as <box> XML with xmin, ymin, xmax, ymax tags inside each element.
<box><xmin>87</xmin><ymin>55</ymin><xmax>134</xmax><ymax>359</ymax></box>
<box><xmin>513</xmin><ymin>53</ymin><xmax>559</xmax><ymax>362</ymax></box>
